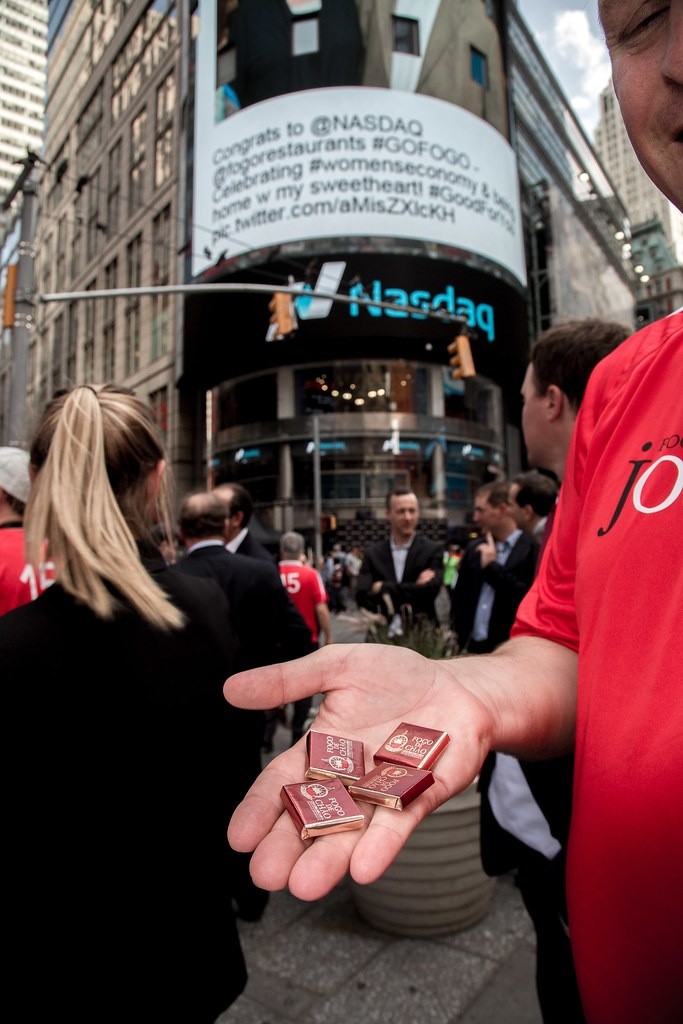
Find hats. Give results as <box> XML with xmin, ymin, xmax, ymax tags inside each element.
<box><xmin>0</xmin><ymin>446</ymin><xmax>32</xmax><ymax>503</ymax></box>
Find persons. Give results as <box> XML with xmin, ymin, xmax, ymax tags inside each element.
<box><xmin>0</xmin><ymin>383</ymin><xmax>248</xmax><ymax>1024</ymax></box>
<box><xmin>261</xmin><ymin>469</ymin><xmax>558</xmax><ymax>754</ymax></box>
<box><xmin>224</xmin><ymin>0</ymin><xmax>683</xmax><ymax>1024</ymax></box>
<box><xmin>0</xmin><ymin>446</ymin><xmax>62</xmax><ymax>617</ymax></box>
<box><xmin>157</xmin><ymin>483</ymin><xmax>314</xmax><ymax>659</ymax></box>
<box><xmin>475</xmin><ymin>319</ymin><xmax>635</xmax><ymax>1024</ymax></box>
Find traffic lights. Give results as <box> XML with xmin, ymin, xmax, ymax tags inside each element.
<box><xmin>321</xmin><ymin>516</ymin><xmax>337</xmax><ymax>531</ymax></box>
<box><xmin>448</xmin><ymin>334</ymin><xmax>476</xmax><ymax>380</ymax></box>
<box><xmin>268</xmin><ymin>292</ymin><xmax>292</xmax><ymax>340</ymax></box>
